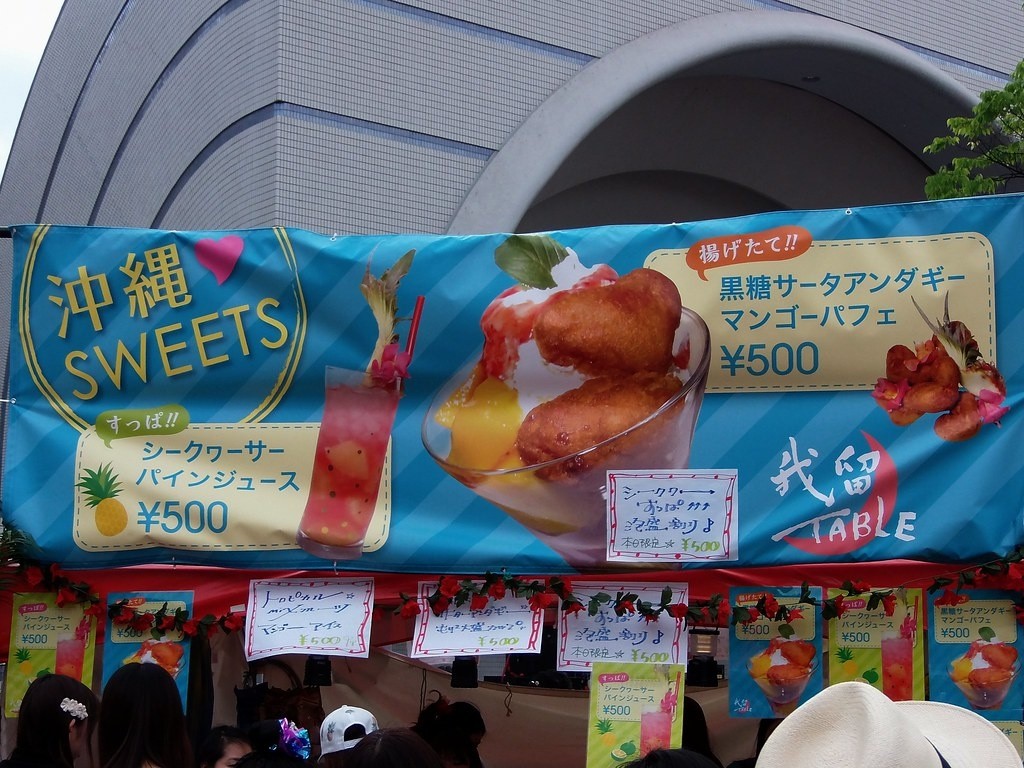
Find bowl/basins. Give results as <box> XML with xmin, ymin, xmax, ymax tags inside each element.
<box><xmin>947</xmin><ymin>653</ymin><xmax>1022</xmax><ymax>710</ymax></box>
<box><xmin>746</xmin><ymin>653</ymin><xmax>818</xmax><ymax>714</ymax></box>
<box><xmin>119</xmin><ymin>649</ymin><xmax>187</xmax><ymax>682</ymax></box>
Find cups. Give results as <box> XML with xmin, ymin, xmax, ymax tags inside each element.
<box><xmin>420</xmin><ymin>307</ymin><xmax>712</xmax><ymax>570</ymax></box>
<box><xmin>55</xmin><ymin>632</ymin><xmax>85</xmax><ymax>682</ymax></box>
<box><xmin>881</xmin><ymin>630</ymin><xmax>914</xmax><ymax>702</ymax></box>
<box><xmin>297</xmin><ymin>365</ymin><xmax>404</xmax><ymax>559</ymax></box>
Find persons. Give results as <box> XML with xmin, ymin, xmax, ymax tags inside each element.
<box><xmin>315</xmin><ymin>703</ymin><xmax>380</xmax><ymax>768</ymax></box>
<box><xmin>406</xmin><ymin>698</ymin><xmax>486</xmax><ymax>768</ymax></box>
<box><xmin>348</xmin><ymin>725</ymin><xmax>438</xmax><ymax>768</ymax></box>
<box><xmin>0</xmin><ymin>673</ymin><xmax>99</xmax><ymax>768</ymax></box>
<box><xmin>722</xmin><ymin>717</ymin><xmax>785</xmax><ymax>768</ymax></box>
<box><xmin>233</xmin><ymin>748</ymin><xmax>304</xmax><ymax>768</ymax></box>
<box><xmin>614</xmin><ymin>746</ymin><xmax>719</xmax><ymax>768</ymax></box>
<box><xmin>679</xmin><ymin>694</ymin><xmax>711</xmax><ymax>760</ymax></box>
<box><xmin>97</xmin><ymin>662</ymin><xmax>199</xmax><ymax>768</ymax></box>
<box><xmin>195</xmin><ymin>724</ymin><xmax>254</xmax><ymax>768</ymax></box>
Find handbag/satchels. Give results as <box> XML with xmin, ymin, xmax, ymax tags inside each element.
<box><xmin>233</xmin><ymin>659</ymin><xmax>325</xmax><ymax>745</ymax></box>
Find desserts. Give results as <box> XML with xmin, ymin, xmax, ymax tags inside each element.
<box><xmin>746</xmin><ymin>624</ymin><xmax>819</xmax><ymax>704</ymax></box>
<box><xmin>947</xmin><ymin>627</ymin><xmax>1021</xmax><ymax>709</ymax></box>
<box><xmin>420</xmin><ymin>236</ymin><xmax>712</xmax><ymax>568</ymax></box>
<box><xmin>118</xmin><ymin>634</ymin><xmax>185</xmax><ymax>681</ymax></box>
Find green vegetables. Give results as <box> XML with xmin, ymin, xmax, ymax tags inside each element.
<box><xmin>361</xmin><ymin>247</ymin><xmax>417</xmax><ymax>387</ymax></box>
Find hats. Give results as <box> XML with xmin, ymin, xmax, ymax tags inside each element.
<box><xmin>754</xmin><ymin>681</ymin><xmax>1023</xmax><ymax>768</ymax></box>
<box><xmin>317</xmin><ymin>704</ymin><xmax>379</xmax><ymax>764</ymax></box>
